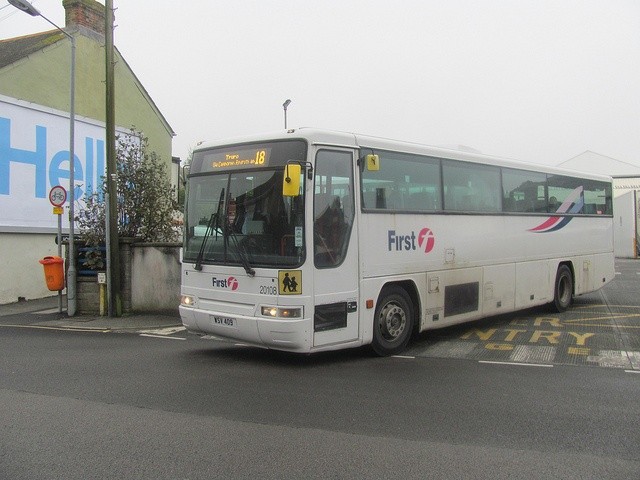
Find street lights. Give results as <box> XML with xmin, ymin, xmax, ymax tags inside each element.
<box><xmin>10</xmin><ymin>1</ymin><xmax>80</xmax><ymax>318</ymax></box>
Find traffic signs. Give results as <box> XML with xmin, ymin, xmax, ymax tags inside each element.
<box><xmin>48</xmin><ymin>186</ymin><xmax>66</xmax><ymax>206</ymax></box>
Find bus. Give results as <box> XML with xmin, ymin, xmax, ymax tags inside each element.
<box><xmin>178</xmin><ymin>129</ymin><xmax>616</xmax><ymax>357</ymax></box>
